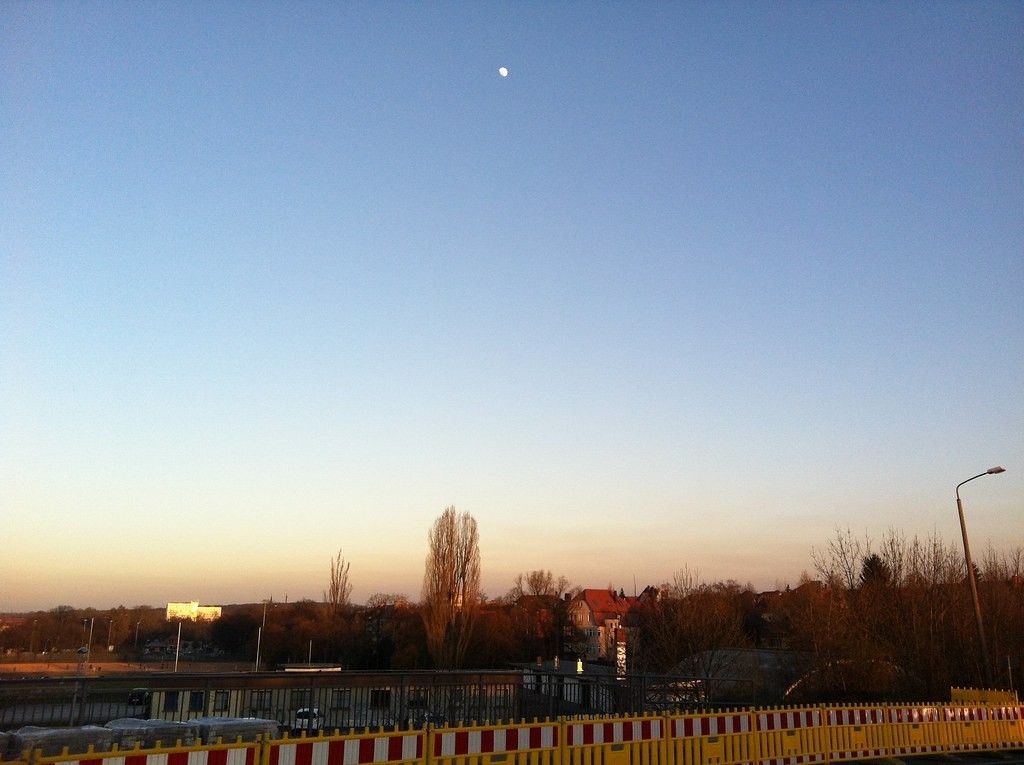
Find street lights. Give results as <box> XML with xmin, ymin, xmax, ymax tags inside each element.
<box><xmin>89</xmin><ymin>618</ymin><xmax>94</xmax><ymax>653</ymax></box>
<box><xmin>135</xmin><ymin>622</ymin><xmax>139</xmax><ymax>649</ymax></box>
<box><xmin>956</xmin><ymin>466</ymin><xmax>1006</xmax><ymax>690</ymax></box>
<box><xmin>70</xmin><ymin>647</ymin><xmax>89</xmax><ymax>728</ymax></box>
<box><xmin>84</xmin><ymin>620</ymin><xmax>87</xmax><ymax>632</ymax></box>
<box><xmin>30</xmin><ymin>620</ymin><xmax>37</xmax><ymax>652</ymax></box>
<box><xmin>107</xmin><ymin>621</ymin><xmax>113</xmax><ymax>653</ymax></box>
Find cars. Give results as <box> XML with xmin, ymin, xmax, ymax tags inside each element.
<box><xmin>297</xmin><ymin>708</ymin><xmax>328</xmax><ymax>730</ymax></box>
